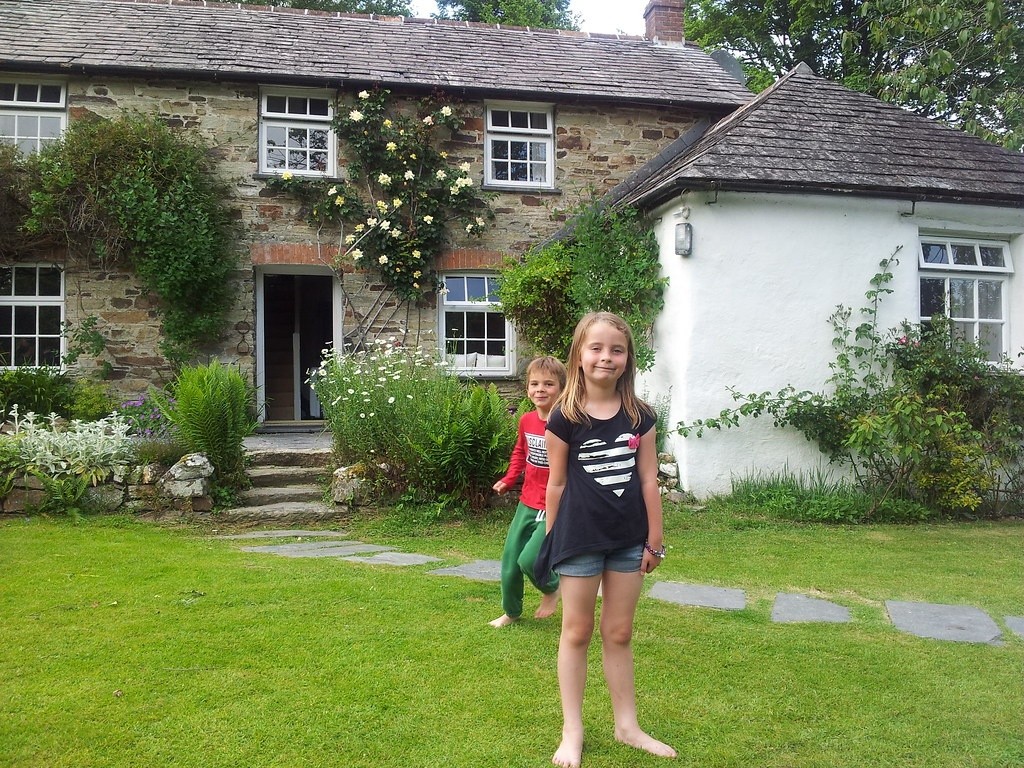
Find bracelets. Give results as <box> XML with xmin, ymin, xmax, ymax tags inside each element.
<box><xmin>645</xmin><ymin>538</ymin><xmax>667</xmax><ymax>559</ymax></box>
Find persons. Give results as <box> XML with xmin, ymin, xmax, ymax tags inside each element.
<box><xmin>545</xmin><ymin>312</ymin><xmax>676</xmax><ymax>768</ymax></box>
<box><xmin>488</xmin><ymin>355</ymin><xmax>568</xmax><ymax>628</ymax></box>
